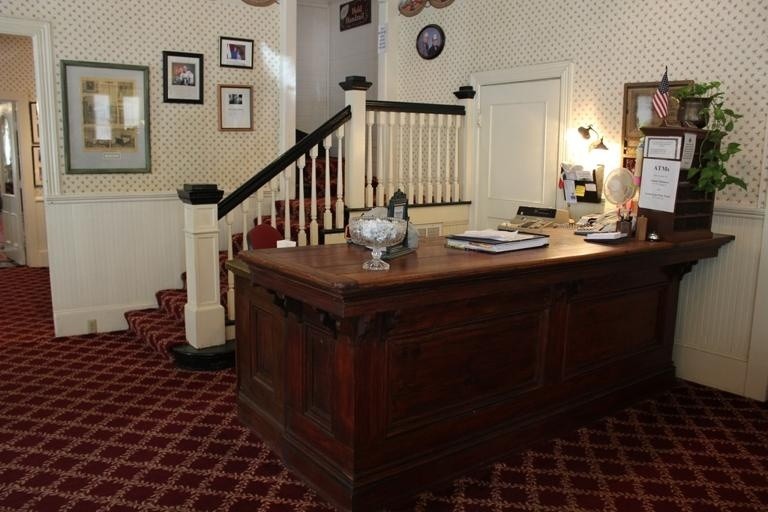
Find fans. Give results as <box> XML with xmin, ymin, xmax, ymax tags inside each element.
<box><xmin>604</xmin><ymin>168</ymin><xmax>636</xmax><ymax>208</ymax></box>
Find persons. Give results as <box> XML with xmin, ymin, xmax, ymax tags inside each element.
<box><xmin>180</xmin><ymin>65</ymin><xmax>194</xmax><ymax>85</ymax></box>
<box><xmin>420</xmin><ymin>32</ymin><xmax>429</xmax><ymax>57</ymax></box>
<box><xmin>428</xmin><ymin>34</ymin><xmax>440</xmax><ymax>57</ymax></box>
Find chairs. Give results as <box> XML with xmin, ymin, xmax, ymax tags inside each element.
<box><xmin>245</xmin><ymin>225</ymin><xmax>287</xmax><ymax>250</ymax></box>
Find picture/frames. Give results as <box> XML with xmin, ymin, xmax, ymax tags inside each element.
<box><xmin>415</xmin><ymin>24</ymin><xmax>445</xmax><ymax>60</ymax></box>
<box><xmin>160</xmin><ymin>35</ymin><xmax>253</xmax><ymax>133</ymax></box>
<box><xmin>60</xmin><ymin>59</ymin><xmax>151</xmax><ymax>174</ymax></box>
<box><xmin>28</xmin><ymin>101</ymin><xmax>42</xmax><ymax>188</ymax></box>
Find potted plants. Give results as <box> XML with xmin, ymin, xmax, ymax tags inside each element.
<box><xmin>671</xmin><ymin>81</ymin><xmax>746</xmax><ymax>194</ymax></box>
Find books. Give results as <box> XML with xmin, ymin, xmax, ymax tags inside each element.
<box><xmin>445</xmin><ymin>230</ymin><xmax>549</xmax><ymax>254</ymax></box>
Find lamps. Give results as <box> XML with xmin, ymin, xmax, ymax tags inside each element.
<box><xmin>578</xmin><ymin>123</ymin><xmax>609</xmax><ymax>152</ymax></box>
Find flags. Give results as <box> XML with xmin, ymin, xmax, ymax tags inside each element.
<box><xmin>653</xmin><ymin>71</ymin><xmax>669</xmax><ymax>119</ymax></box>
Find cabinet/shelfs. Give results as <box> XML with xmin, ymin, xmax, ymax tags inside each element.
<box><xmin>640</xmin><ymin>133</ymin><xmax>720</xmax><ymax>241</ymax></box>
